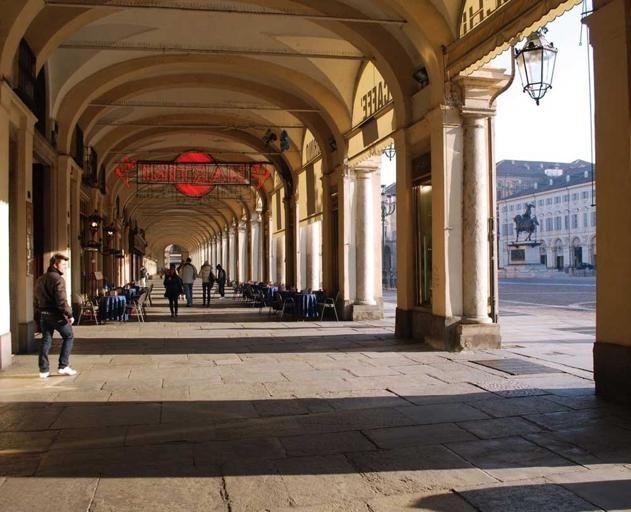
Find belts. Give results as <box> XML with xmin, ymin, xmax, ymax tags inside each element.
<box><xmin>41</xmin><ymin>311</ymin><xmax>51</xmax><ymax>315</ymax></box>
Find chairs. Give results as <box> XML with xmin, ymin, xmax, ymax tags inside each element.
<box><xmin>231</xmin><ymin>279</ymin><xmax>344</xmax><ymax>324</ymax></box>
<box><xmin>73</xmin><ymin>279</ymin><xmax>156</xmax><ymax>325</ymax></box>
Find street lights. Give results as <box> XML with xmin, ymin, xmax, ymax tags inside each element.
<box><xmin>380</xmin><ymin>184</ymin><xmax>395</xmax><ymax>283</ymax></box>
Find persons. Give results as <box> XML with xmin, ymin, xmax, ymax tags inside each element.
<box><xmin>33</xmin><ymin>253</ymin><xmax>77</xmax><ymax>378</ymax></box>
<box><xmin>123</xmin><ymin>280</ymin><xmax>139</xmax><ymax>303</ymax></box>
<box><xmin>162</xmin><ymin>266</ymin><xmax>185</xmax><ymax>317</ymax></box>
<box><xmin>176</xmin><ymin>258</ymin><xmax>226</xmax><ymax>307</ymax></box>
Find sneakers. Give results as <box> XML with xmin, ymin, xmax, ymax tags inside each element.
<box><xmin>39</xmin><ymin>371</ymin><xmax>49</xmax><ymax>379</ymax></box>
<box><xmin>170</xmin><ymin>294</ymin><xmax>224</xmax><ymax>318</ymax></box>
<box><xmin>58</xmin><ymin>367</ymin><xmax>76</xmax><ymax>375</ymax></box>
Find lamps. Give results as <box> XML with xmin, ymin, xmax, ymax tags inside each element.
<box><xmin>80</xmin><ymin>208</ymin><xmax>104</xmax><ymax>253</ymax></box>
<box><xmin>97</xmin><ymin>221</ymin><xmax>119</xmax><ymax>257</ymax></box>
<box><xmin>512</xmin><ymin>25</ymin><xmax>559</xmax><ymax>106</ymax></box>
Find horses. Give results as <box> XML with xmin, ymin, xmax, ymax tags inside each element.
<box><xmin>513</xmin><ymin>214</ymin><xmax>539</xmax><ymax>242</ymax></box>
